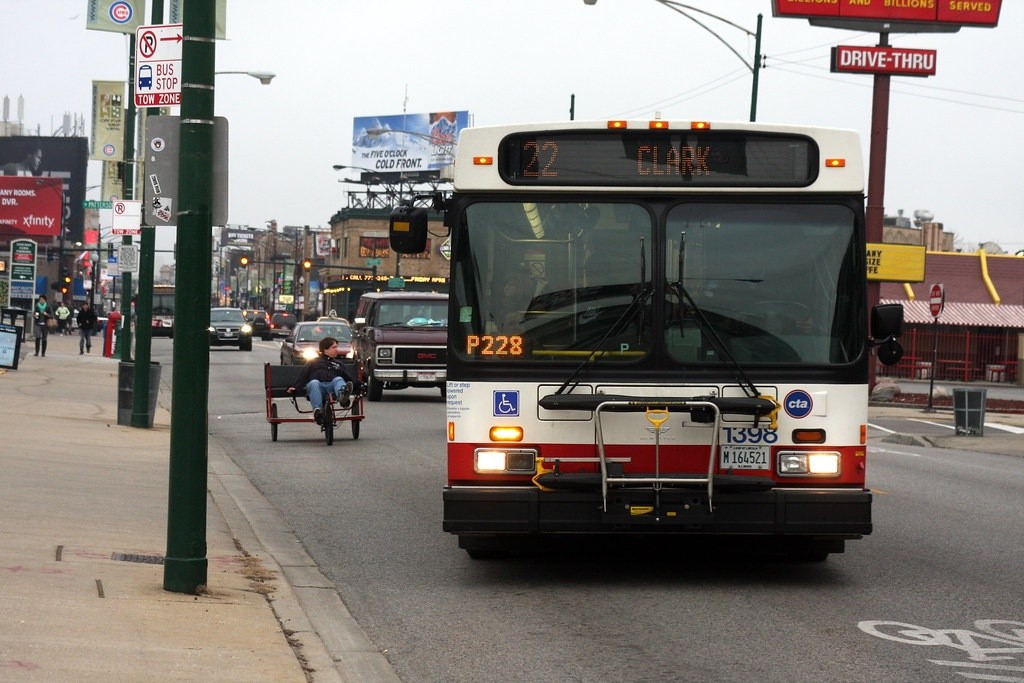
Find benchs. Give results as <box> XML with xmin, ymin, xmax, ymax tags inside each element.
<box><xmin>264</xmin><ymin>358</ymin><xmax>365</xmax><ymax>423</ymax></box>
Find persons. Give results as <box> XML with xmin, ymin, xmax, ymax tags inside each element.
<box><xmin>34</xmin><ymin>295</ymin><xmax>54</xmax><ymax>357</ymax></box>
<box><xmin>287</xmin><ymin>337</ymin><xmax>357</xmax><ymax>425</ymax></box>
<box><xmin>55</xmin><ymin>302</ymin><xmax>70</xmax><ymax>335</ymax></box>
<box><xmin>492</xmin><ymin>272</ymin><xmax>533</xmax><ymax>331</ymax></box>
<box><xmin>3</xmin><ymin>146</ymin><xmax>42</xmax><ymax>176</ymax></box>
<box><xmin>50</xmin><ymin>298</ymin><xmax>59</xmax><ymax>335</ymax></box>
<box><xmin>77</xmin><ymin>302</ymin><xmax>97</xmax><ymax>355</ymax></box>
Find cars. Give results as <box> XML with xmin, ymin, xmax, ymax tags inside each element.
<box><xmin>66</xmin><ymin>308</ymin><xmax>80</xmax><ymax>331</ymax></box>
<box><xmin>280</xmin><ymin>321</ymin><xmax>358</xmax><ymax>362</ymax></box>
<box><xmin>315</xmin><ymin>309</ymin><xmax>351</xmax><ymax>335</ymax></box>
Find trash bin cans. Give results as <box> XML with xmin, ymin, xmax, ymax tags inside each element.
<box><xmin>113</xmin><ymin>329</ymin><xmax>133</xmax><ymax>359</ymax></box>
<box><xmin>117</xmin><ymin>359</ymin><xmax>163</xmax><ymax>427</ymax></box>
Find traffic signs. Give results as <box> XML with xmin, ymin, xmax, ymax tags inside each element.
<box><xmin>136</xmin><ymin>24</ymin><xmax>183</xmax><ymax>108</ymax></box>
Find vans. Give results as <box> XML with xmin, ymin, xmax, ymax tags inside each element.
<box><xmin>352</xmin><ymin>292</ymin><xmax>450</xmax><ymax>403</ymax></box>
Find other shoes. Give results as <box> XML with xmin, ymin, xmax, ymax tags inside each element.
<box><xmin>87</xmin><ymin>346</ymin><xmax>90</xmax><ymax>352</ymax></box>
<box><xmin>79</xmin><ymin>351</ymin><xmax>84</xmax><ymax>355</ymax></box>
<box><xmin>339</xmin><ymin>382</ymin><xmax>353</xmax><ymax>406</ymax></box>
<box><xmin>313</xmin><ymin>408</ymin><xmax>324</xmax><ymax>424</ymax></box>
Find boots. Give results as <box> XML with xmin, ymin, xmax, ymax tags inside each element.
<box><xmin>42</xmin><ymin>340</ymin><xmax>46</xmax><ymax>357</ymax></box>
<box><xmin>34</xmin><ymin>337</ymin><xmax>40</xmax><ymax>356</ymax></box>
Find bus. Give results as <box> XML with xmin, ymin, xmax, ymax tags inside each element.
<box><xmin>388</xmin><ymin>121</ymin><xmax>905</xmax><ymax>572</ymax></box>
<box><xmin>134</xmin><ymin>284</ymin><xmax>175</xmax><ymax>341</ymax></box>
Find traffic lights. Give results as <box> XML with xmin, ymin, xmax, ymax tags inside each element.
<box><xmin>62</xmin><ymin>267</ymin><xmax>71</xmax><ymax>294</ymax></box>
<box><xmin>304</xmin><ymin>257</ymin><xmax>311</xmax><ymax>272</ymax></box>
<box><xmin>241</xmin><ymin>253</ymin><xmax>248</xmax><ymax>269</ymax></box>
<box><xmin>47</xmin><ymin>246</ymin><xmax>54</xmax><ymax>262</ymax></box>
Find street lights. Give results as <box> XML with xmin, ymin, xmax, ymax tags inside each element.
<box><xmin>213</xmin><ymin>226</ymin><xmax>298</xmax><ymax>314</ymax></box>
<box><xmin>88</xmin><ymin>250</ymin><xmax>99</xmax><ymax>329</ymax></box>
<box><xmin>583</xmin><ymin>0</ymin><xmax>766</xmax><ymax>122</ymax></box>
<box><xmin>334</xmin><ymin>164</ymin><xmax>403</xmax><ymax>278</ymax></box>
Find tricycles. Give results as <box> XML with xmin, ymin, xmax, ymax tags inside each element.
<box><xmin>263</xmin><ymin>361</ymin><xmax>366</xmax><ymax>448</ymax></box>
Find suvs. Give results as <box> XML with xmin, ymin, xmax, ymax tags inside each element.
<box><xmin>243</xmin><ymin>308</ymin><xmax>271</xmax><ymax>341</ymax></box>
<box><xmin>209</xmin><ymin>307</ymin><xmax>253</xmax><ymax>351</ymax></box>
<box><xmin>270</xmin><ymin>313</ymin><xmax>298</xmax><ymax>341</ymax></box>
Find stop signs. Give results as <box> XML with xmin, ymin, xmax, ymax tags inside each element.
<box><xmin>928</xmin><ymin>284</ymin><xmax>945</xmax><ymax>319</ymax></box>
